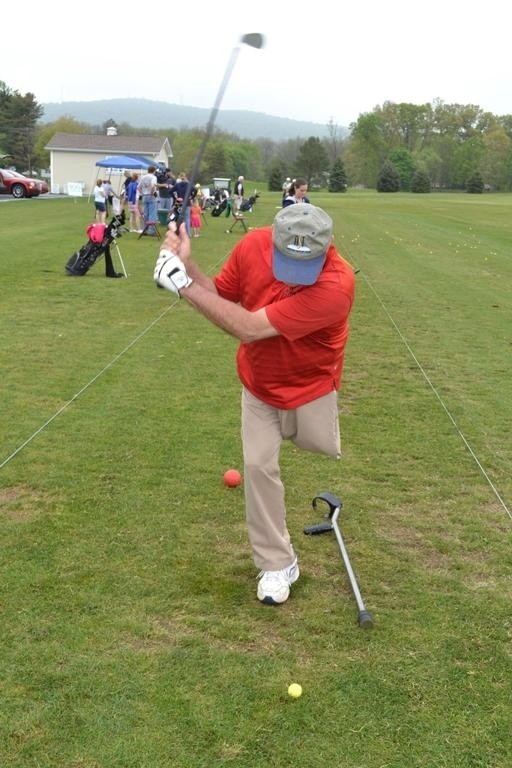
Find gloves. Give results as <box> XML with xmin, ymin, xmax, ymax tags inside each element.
<box><xmin>154</xmin><ymin>249</ymin><xmax>195</xmax><ymax>299</ymax></box>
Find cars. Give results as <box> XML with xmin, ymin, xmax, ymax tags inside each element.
<box><xmin>0</xmin><ymin>166</ymin><xmax>49</xmax><ymax>200</ymax></box>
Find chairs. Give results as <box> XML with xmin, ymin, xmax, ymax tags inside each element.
<box><xmin>137</xmin><ymin>206</ymin><xmax>161</xmax><ymax>241</ymax></box>
<box><xmin>230</xmin><ymin>205</ymin><xmax>248</xmax><ymax>233</ymax></box>
<box><xmin>190</xmin><ymin>199</ymin><xmax>208</xmax><ymax>225</ymax></box>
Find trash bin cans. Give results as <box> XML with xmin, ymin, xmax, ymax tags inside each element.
<box><xmin>157</xmin><ymin>208</ymin><xmax>169</xmax><ymax>225</ymax></box>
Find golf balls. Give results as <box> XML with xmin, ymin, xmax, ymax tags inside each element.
<box><xmin>223</xmin><ymin>469</ymin><xmax>241</xmax><ymax>488</ymax></box>
<box><xmin>286</xmin><ymin>682</ymin><xmax>304</xmax><ymax>698</ymax></box>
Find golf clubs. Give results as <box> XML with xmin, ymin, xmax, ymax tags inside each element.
<box><xmin>174</xmin><ymin>32</ymin><xmax>262</xmax><ymax>234</ymax></box>
<box><xmin>110</xmin><ymin>215</ymin><xmax>131</xmax><ymax>241</ymax></box>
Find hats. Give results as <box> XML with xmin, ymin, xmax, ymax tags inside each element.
<box><xmin>272</xmin><ymin>202</ymin><xmax>334</xmax><ymax>285</ymax></box>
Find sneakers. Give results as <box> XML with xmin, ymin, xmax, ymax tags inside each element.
<box><xmin>256</xmin><ymin>557</ymin><xmax>297</xmax><ymax>605</ymax></box>
<box><xmin>92</xmin><ymin>222</ymin><xmax>202</xmax><ymax>240</ymax></box>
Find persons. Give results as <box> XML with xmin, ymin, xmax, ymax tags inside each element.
<box><xmin>123</xmin><ymin>166</ymin><xmax>203</xmax><ymax>238</ymax></box>
<box><xmin>233</xmin><ymin>174</ymin><xmax>244</xmax><ymax>215</ymax></box>
<box><xmin>149</xmin><ymin>202</ymin><xmax>355</xmax><ymax>605</ymax></box>
<box><xmin>93</xmin><ymin>179</ymin><xmax>109</xmax><ymax>227</ymax></box>
<box><xmin>103</xmin><ymin>180</ymin><xmax>120</xmax><ymax>205</ymax></box>
<box><xmin>287</xmin><ymin>178</ymin><xmax>296</xmax><ymax>196</ymax></box>
<box><xmin>281</xmin><ymin>177</ymin><xmax>291</xmax><ymax>196</ymax></box>
<box><xmin>283</xmin><ymin>177</ymin><xmax>310</xmax><ymax>207</ymax></box>
